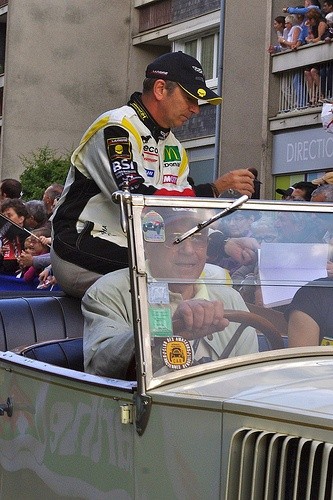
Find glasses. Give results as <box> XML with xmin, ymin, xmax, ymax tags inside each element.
<box><xmin>163</xmin><ymin>233</ymin><xmax>210</xmax><ymax>251</ymax></box>
<box><xmin>281</xmin><ymin>195</ymin><xmax>287</xmax><ymax>199</ymax></box>
<box><xmin>257</xmin><ymin>237</ymin><xmax>275</xmax><ymax>243</ymax></box>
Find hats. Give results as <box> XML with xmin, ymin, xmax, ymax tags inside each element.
<box><xmin>146</xmin><ymin>205</ymin><xmax>209</xmax><ymax>237</ymax></box>
<box><xmin>276</xmin><ymin>182</ymin><xmax>299</xmax><ymax>196</ymax></box>
<box><xmin>145</xmin><ymin>50</ymin><xmax>224</xmax><ymax>105</ymax></box>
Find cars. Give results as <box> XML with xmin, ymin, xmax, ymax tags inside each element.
<box><xmin>1</xmin><ymin>188</ymin><xmax>333</xmax><ymax>500</ymax></box>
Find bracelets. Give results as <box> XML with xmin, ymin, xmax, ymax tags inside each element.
<box><xmin>223</xmin><ymin>236</ymin><xmax>231</xmax><ymax>258</ymax></box>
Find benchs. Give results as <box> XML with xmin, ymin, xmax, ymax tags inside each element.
<box><xmin>0</xmin><ymin>290</ymin><xmax>84</xmax><ymax>375</ymax></box>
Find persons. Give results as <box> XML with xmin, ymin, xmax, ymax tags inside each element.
<box><xmin>268</xmin><ymin>0</ymin><xmax>333</xmax><ymax>113</ymax></box>
<box><xmin>49</xmin><ymin>51</ymin><xmax>255</xmax><ymax>299</ymax></box>
<box><xmin>82</xmin><ymin>207</ymin><xmax>260</xmax><ymax>382</ymax></box>
<box><xmin>0</xmin><ymin>178</ymin><xmax>64</xmax><ymax>290</ymax></box>
<box><xmin>187</xmin><ymin>168</ymin><xmax>333</xmax><ymax>348</ymax></box>
